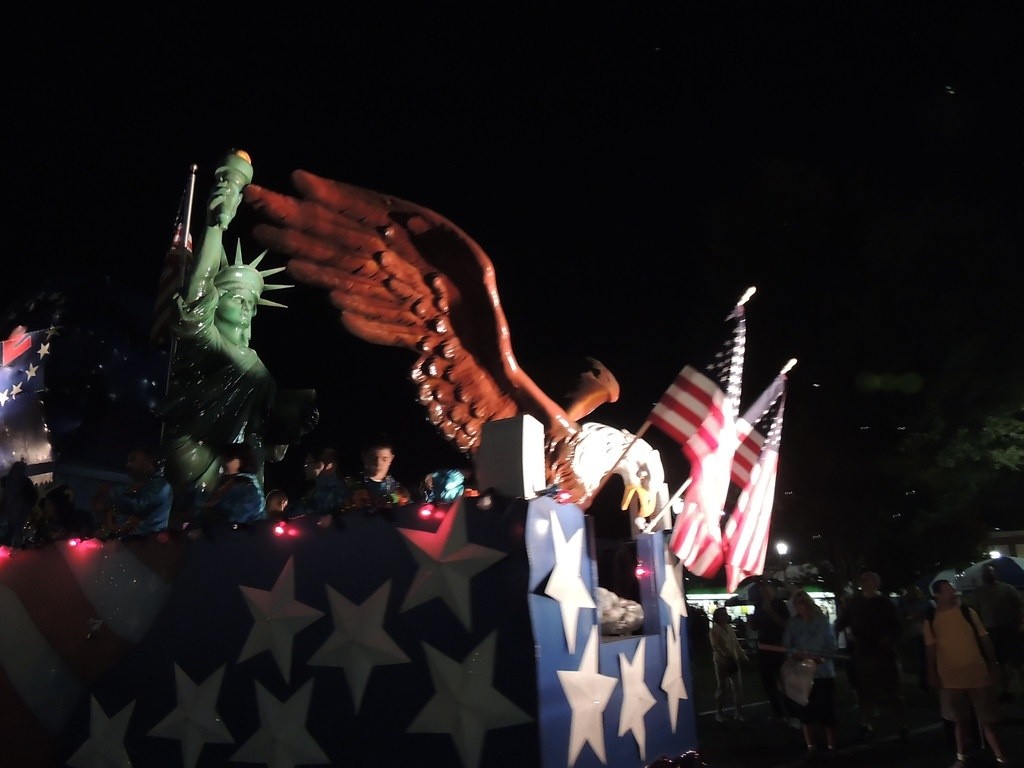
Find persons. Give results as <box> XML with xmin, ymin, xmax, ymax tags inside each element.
<box><xmin>0</xmin><ymin>444</ymin><xmax>465</xmax><ymax>540</ymax></box>
<box><xmin>748</xmin><ymin>578</ymin><xmax>802</xmax><ymax>729</ymax></box>
<box><xmin>783</xmin><ymin>590</ymin><xmax>840</xmax><ymax>766</ymax></box>
<box><xmin>708</xmin><ymin>608</ymin><xmax>751</xmax><ymax>729</ymax></box>
<box><xmin>979</xmin><ymin>565</ymin><xmax>1024</xmax><ymax>703</ymax></box>
<box><xmin>906</xmin><ymin>579</ymin><xmax>1009</xmax><ymax>768</ymax></box>
<box><xmin>832</xmin><ymin>571</ymin><xmax>911</xmax><ymax>745</ymax></box>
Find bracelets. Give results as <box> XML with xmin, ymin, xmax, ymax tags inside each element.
<box><xmin>989</xmin><ymin>656</ymin><xmax>1001</xmax><ymax>665</ymax></box>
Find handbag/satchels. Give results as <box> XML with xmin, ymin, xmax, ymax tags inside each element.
<box><xmin>775</xmin><ymin>659</ymin><xmax>813</xmax><ymax>707</ymax></box>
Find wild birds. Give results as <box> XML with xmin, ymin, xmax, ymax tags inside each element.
<box><xmin>238</xmin><ymin>168</ymin><xmax>619</xmax><ymax>504</ymax></box>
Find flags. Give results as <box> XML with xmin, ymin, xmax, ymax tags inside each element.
<box><xmin>647</xmin><ymin>327</ymin><xmax>747</xmax><ymax>579</ymax></box>
<box><xmin>721</xmin><ymin>383</ymin><xmax>786</xmax><ymax>595</ymax></box>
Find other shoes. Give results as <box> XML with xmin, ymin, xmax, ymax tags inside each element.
<box><xmin>733</xmin><ymin>712</ymin><xmax>746</xmax><ymax>721</ymax></box>
<box><xmin>995</xmin><ymin>754</ymin><xmax>1009</xmax><ymax>768</ymax></box>
<box><xmin>861</xmin><ymin>724</ymin><xmax>873</xmax><ymax>736</ymax></box>
<box><xmin>826</xmin><ymin>745</ymin><xmax>836</xmax><ymax>759</ymax></box>
<box><xmin>714</xmin><ymin>712</ymin><xmax>727</xmax><ymax>722</ymax></box>
<box><xmin>895</xmin><ymin>723</ymin><xmax>910</xmax><ymax>737</ymax></box>
<box><xmin>956</xmin><ymin>752</ymin><xmax>970</xmax><ymax>768</ymax></box>
<box><xmin>804</xmin><ymin>746</ymin><xmax>817</xmax><ymax>760</ymax></box>
